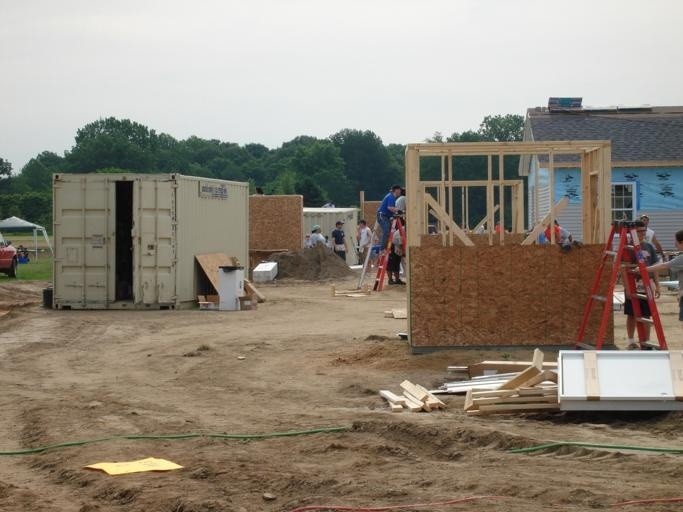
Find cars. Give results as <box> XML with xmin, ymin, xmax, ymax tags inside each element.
<box><xmin>1</xmin><ymin>231</ymin><xmax>17</xmax><ymax>277</ymax></box>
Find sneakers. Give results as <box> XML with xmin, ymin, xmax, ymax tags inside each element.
<box><xmin>388</xmin><ymin>279</ymin><xmax>406</xmax><ymax>284</ymax></box>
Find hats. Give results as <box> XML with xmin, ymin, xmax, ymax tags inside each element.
<box><xmin>336</xmin><ymin>221</ymin><xmax>345</xmax><ymax>225</ymax></box>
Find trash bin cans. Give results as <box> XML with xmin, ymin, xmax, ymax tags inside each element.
<box><xmin>219</xmin><ymin>266</ymin><xmax>245</xmax><ymax>310</ymax></box>
<box><xmin>42</xmin><ymin>289</ymin><xmax>52</xmax><ymax>306</ymax></box>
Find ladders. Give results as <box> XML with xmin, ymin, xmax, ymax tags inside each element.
<box><xmin>573</xmin><ymin>219</ymin><xmax>668</xmax><ymax>351</ymax></box>
<box><xmin>358</xmin><ymin>213</ymin><xmax>406</xmax><ymax>291</ymax></box>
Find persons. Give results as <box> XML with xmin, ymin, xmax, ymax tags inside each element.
<box><xmin>622</xmin><ymin>211</ymin><xmax>668</xmax><ymax>264</ymax></box>
<box><xmin>554</xmin><ymin>219</ymin><xmax>573</xmax><ymax>243</ymax></box>
<box><xmin>0</xmin><ymin>242</ymin><xmax>8</xmax><ymax>251</ymax></box>
<box><xmin>331</xmin><ymin>220</ymin><xmax>349</xmax><ymax>264</ymax></box>
<box><xmin>616</xmin><ymin>219</ymin><xmax>660</xmax><ymax>350</ymax></box>
<box><xmin>358</xmin><ymin>218</ymin><xmax>373</xmax><ymax>271</ymax></box>
<box><xmin>325</xmin><ymin>234</ymin><xmax>332</xmax><ymax>250</ymax></box>
<box><xmin>375</xmin><ymin>184</ymin><xmax>403</xmax><ymax>255</ymax></box>
<box><xmin>322</xmin><ymin>200</ymin><xmax>335</xmax><ymax>208</ymax></box>
<box><xmin>635</xmin><ymin>229</ymin><xmax>683</xmax><ymax>322</ymax></box>
<box><xmin>18</xmin><ymin>244</ymin><xmax>29</xmax><ymax>258</ymax></box>
<box><xmin>8</xmin><ymin>241</ymin><xmax>19</xmax><ymax>266</ymax></box>
<box><xmin>544</xmin><ymin>223</ymin><xmax>561</xmax><ymax>243</ymax></box>
<box><xmin>395</xmin><ymin>187</ymin><xmax>406</xmax><ymax>212</ymax></box>
<box><xmin>310</xmin><ymin>225</ymin><xmax>326</xmax><ymax>248</ymax></box>
<box><xmin>386</xmin><ymin>225</ymin><xmax>406</xmax><ymax>285</ymax></box>
<box><xmin>496</xmin><ymin>221</ymin><xmax>507</xmax><ymax>233</ymax></box>
<box><xmin>303</xmin><ymin>233</ymin><xmax>312</xmax><ymax>248</ymax></box>
